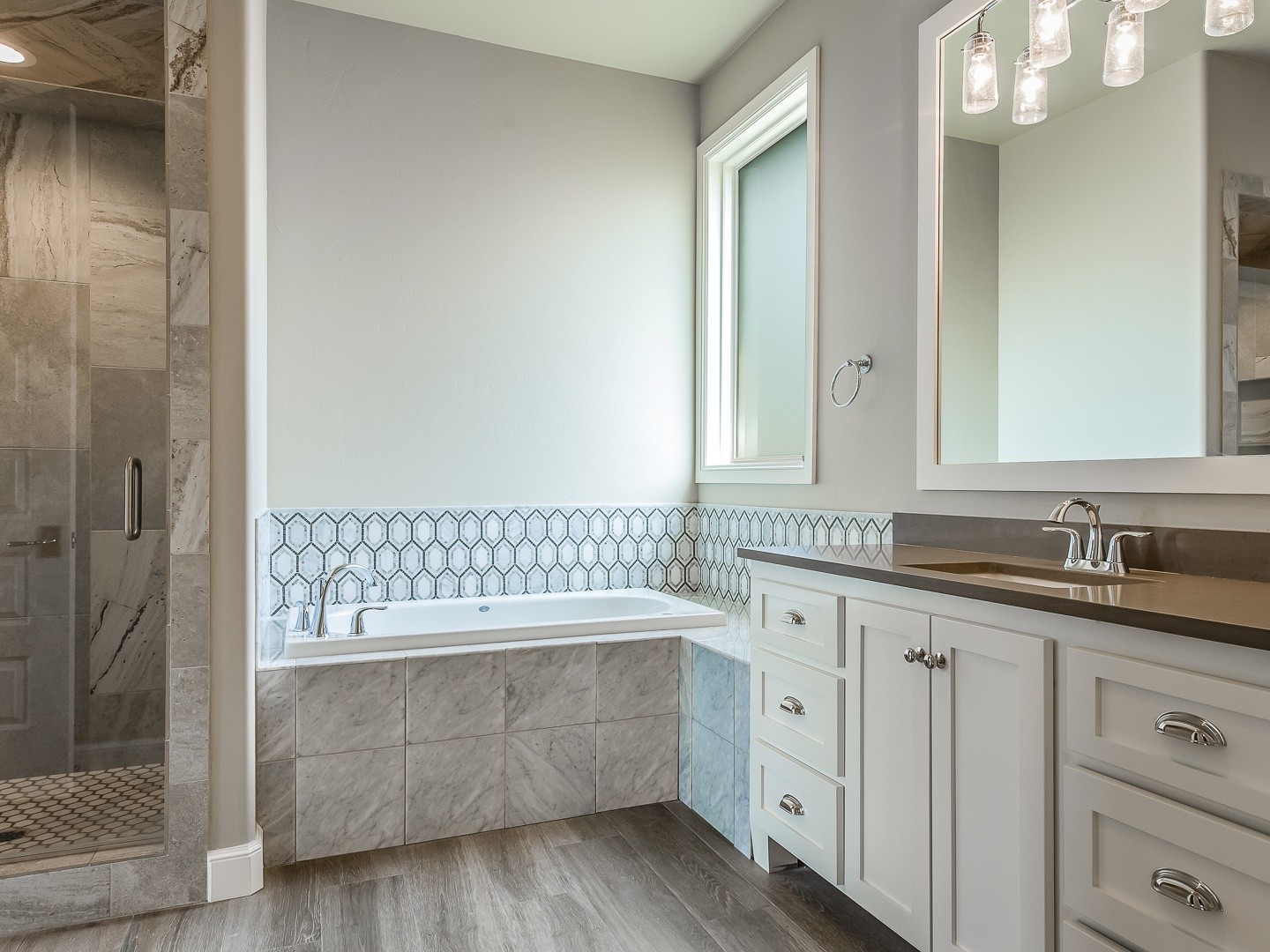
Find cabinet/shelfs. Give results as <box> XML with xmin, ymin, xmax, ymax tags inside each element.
<box><xmin>1060</xmin><ymin>613</ymin><xmax>1270</xmax><ymax>952</ymax></box>
<box><xmin>749</xmin><ymin>558</ymin><xmax>845</xmax><ymax>889</ymax></box>
<box><xmin>845</xmin><ymin>575</ymin><xmax>1060</xmax><ymax>952</ymax></box>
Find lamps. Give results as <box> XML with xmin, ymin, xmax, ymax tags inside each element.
<box><xmin>1012</xmin><ymin>0</ymin><xmax>1255</xmax><ymax>125</ymax></box>
<box><xmin>962</xmin><ymin>0</ymin><xmax>1170</xmax><ymax>115</ymax></box>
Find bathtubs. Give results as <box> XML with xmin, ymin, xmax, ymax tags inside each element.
<box><xmin>898</xmin><ymin>561</ymin><xmax>1167</xmax><ymax>589</ymax></box>
<box><xmin>281</xmin><ymin>587</ymin><xmax>730</xmax><ymax>868</ymax></box>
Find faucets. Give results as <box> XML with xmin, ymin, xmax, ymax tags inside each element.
<box><xmin>1046</xmin><ymin>497</ymin><xmax>1106</xmax><ymax>562</ymax></box>
<box><xmin>307</xmin><ymin>563</ymin><xmax>379</xmax><ymax>639</ymax></box>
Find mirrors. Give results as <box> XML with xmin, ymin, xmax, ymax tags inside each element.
<box><xmin>1237</xmin><ymin>194</ymin><xmax>1269</xmax><ymax>455</ymax></box>
<box><xmin>0</xmin><ymin>0</ymin><xmax>167</xmax><ymax>863</ymax></box>
<box><xmin>915</xmin><ymin>0</ymin><xmax>1270</xmax><ymax>495</ymax></box>
